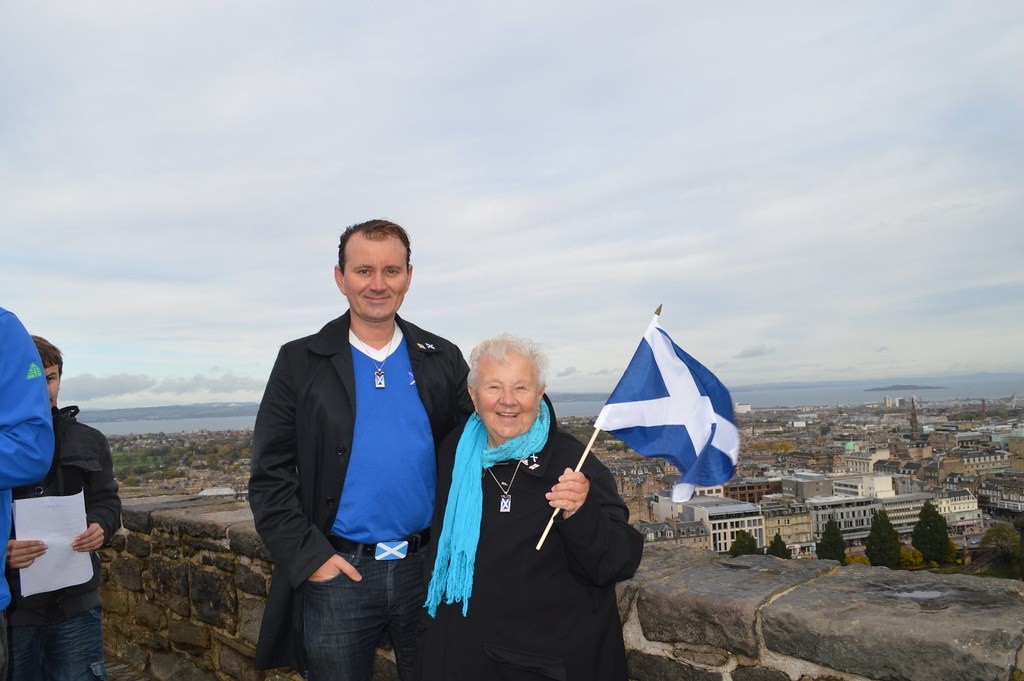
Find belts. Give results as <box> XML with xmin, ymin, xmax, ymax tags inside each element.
<box><xmin>328</xmin><ymin>525</ymin><xmax>431</xmax><ymax>561</ymax></box>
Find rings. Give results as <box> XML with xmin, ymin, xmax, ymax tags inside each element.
<box><xmin>567</xmin><ymin>503</ymin><xmax>575</xmax><ymax>512</ymax></box>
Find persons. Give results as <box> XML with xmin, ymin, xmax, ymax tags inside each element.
<box><xmin>422</xmin><ymin>336</ymin><xmax>644</xmax><ymax>681</ymax></box>
<box><xmin>247</xmin><ymin>220</ymin><xmax>474</xmax><ymax>681</ymax></box>
<box><xmin>0</xmin><ymin>306</ymin><xmax>54</xmax><ymax>681</ymax></box>
<box><xmin>6</xmin><ymin>335</ymin><xmax>122</xmax><ymax>681</ymax></box>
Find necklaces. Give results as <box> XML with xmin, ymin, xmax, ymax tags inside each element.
<box><xmin>488</xmin><ymin>459</ymin><xmax>522</xmax><ymax>512</ymax></box>
<box><xmin>350</xmin><ymin>323</ymin><xmax>395</xmax><ymax>388</ymax></box>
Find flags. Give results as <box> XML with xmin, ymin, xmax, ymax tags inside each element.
<box><xmin>594</xmin><ymin>323</ymin><xmax>739</xmax><ymax>503</ymax></box>
